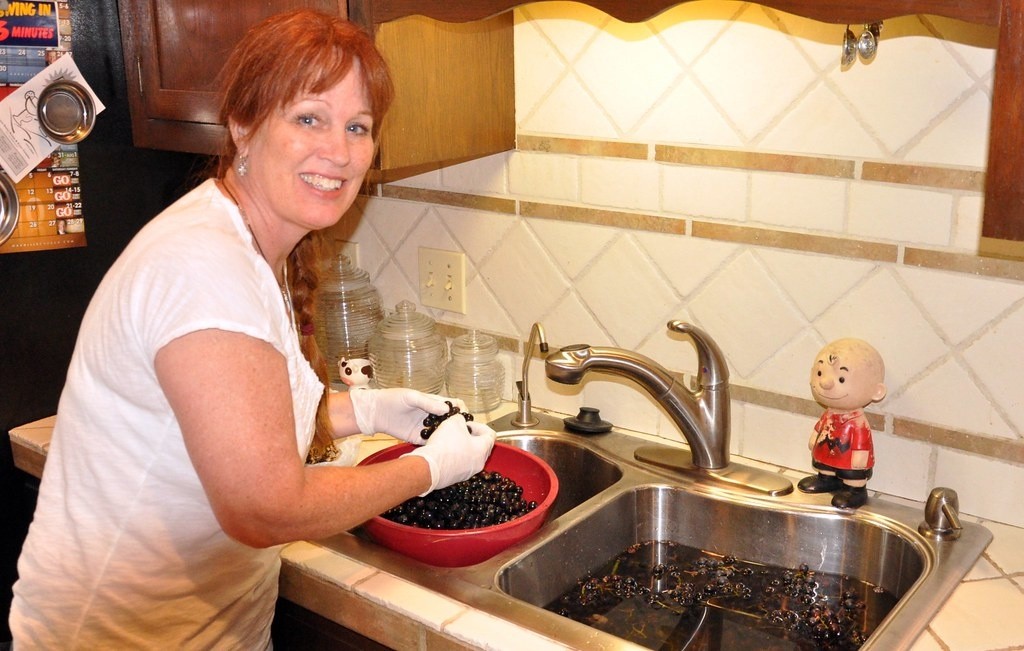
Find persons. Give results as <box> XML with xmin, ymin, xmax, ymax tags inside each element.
<box><xmin>798</xmin><ymin>337</ymin><xmax>886</xmax><ymax>510</ymax></box>
<box><xmin>8</xmin><ymin>10</ymin><xmax>497</xmax><ymax>651</ymax></box>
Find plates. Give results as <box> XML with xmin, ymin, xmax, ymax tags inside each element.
<box><xmin>38</xmin><ymin>80</ymin><xmax>97</xmax><ymax>144</ymax></box>
<box><xmin>0</xmin><ymin>170</ymin><xmax>20</xmax><ymax>246</ymax></box>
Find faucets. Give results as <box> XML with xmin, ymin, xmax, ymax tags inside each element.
<box><xmin>544</xmin><ymin>320</ymin><xmax>729</xmax><ymax>470</ymax></box>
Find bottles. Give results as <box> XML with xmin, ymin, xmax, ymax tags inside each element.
<box><xmin>312</xmin><ymin>252</ymin><xmax>384</xmax><ymax>385</ymax></box>
<box><xmin>444</xmin><ymin>328</ymin><xmax>505</xmax><ymax>413</ymax></box>
<box><xmin>367</xmin><ymin>299</ymin><xmax>449</xmax><ymax>395</ymax></box>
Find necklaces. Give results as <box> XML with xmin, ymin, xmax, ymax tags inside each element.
<box><xmin>222</xmin><ymin>178</ymin><xmax>290</xmax><ymax>313</ymax></box>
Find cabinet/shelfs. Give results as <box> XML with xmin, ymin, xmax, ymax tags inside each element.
<box><xmin>119</xmin><ymin>0</ymin><xmax>515</xmax><ymax>185</ymax></box>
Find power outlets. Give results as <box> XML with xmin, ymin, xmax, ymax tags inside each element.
<box><xmin>334</xmin><ymin>238</ymin><xmax>360</xmax><ymax>269</ymax></box>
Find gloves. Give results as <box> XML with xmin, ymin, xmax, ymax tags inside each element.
<box><xmin>399</xmin><ymin>414</ymin><xmax>497</xmax><ymax>497</ymax></box>
<box><xmin>349</xmin><ymin>385</ymin><xmax>469</xmax><ymax>445</ymax></box>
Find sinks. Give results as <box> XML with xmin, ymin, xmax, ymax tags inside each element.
<box><xmin>342</xmin><ymin>432</ymin><xmax>624</xmax><ymax>598</ymax></box>
<box><xmin>454</xmin><ymin>484</ymin><xmax>936</xmax><ymax>651</ymax></box>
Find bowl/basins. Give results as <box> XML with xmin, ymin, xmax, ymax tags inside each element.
<box><xmin>357</xmin><ymin>440</ymin><xmax>559</xmax><ymax>567</ymax></box>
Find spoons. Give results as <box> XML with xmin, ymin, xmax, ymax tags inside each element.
<box><xmin>840</xmin><ymin>21</ymin><xmax>884</xmax><ymax>71</ymax></box>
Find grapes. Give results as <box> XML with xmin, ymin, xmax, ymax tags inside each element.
<box><xmin>419</xmin><ymin>401</ymin><xmax>474</xmax><ymax>439</ymax></box>
<box><xmin>549</xmin><ymin>551</ymin><xmax>871</xmax><ymax>651</ymax></box>
<box><xmin>384</xmin><ymin>471</ymin><xmax>538</xmax><ymax>530</ymax></box>
